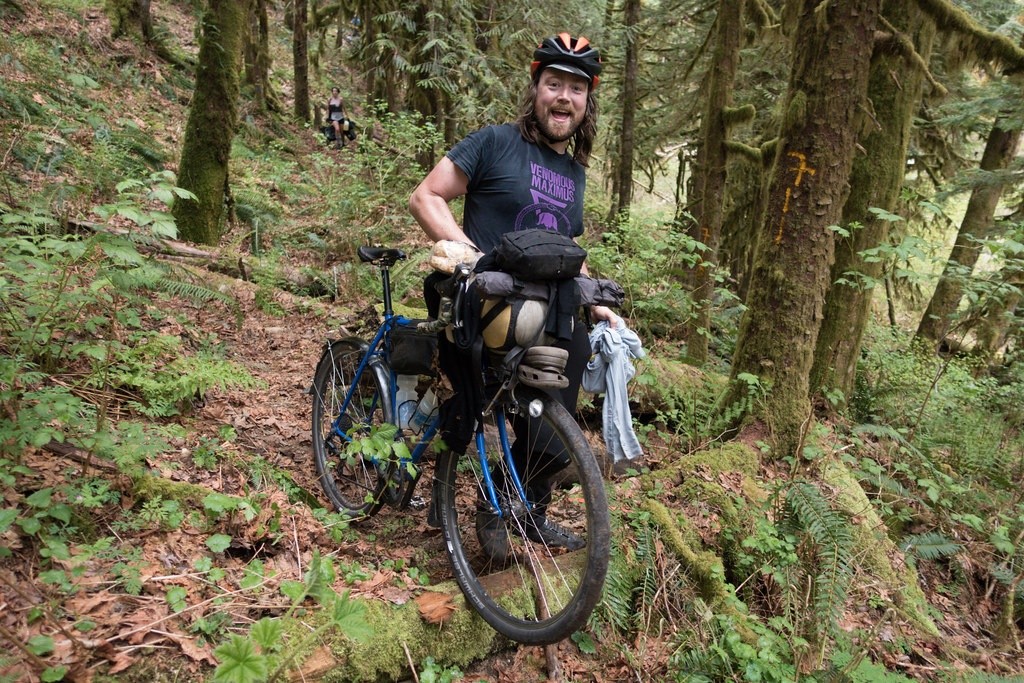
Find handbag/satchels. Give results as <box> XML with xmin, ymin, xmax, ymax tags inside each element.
<box><xmin>495</xmin><ymin>229</ymin><xmax>587</xmax><ymax>280</ymax></box>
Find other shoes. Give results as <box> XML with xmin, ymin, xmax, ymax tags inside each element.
<box><xmin>512</xmin><ymin>518</ymin><xmax>586</xmax><ymax>552</ymax></box>
<box><xmin>476</xmin><ymin>479</ymin><xmax>510</xmax><ymax>563</ymax></box>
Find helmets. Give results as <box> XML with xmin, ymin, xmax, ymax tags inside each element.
<box><xmin>530</xmin><ymin>32</ymin><xmax>603</xmax><ymax>92</ymax></box>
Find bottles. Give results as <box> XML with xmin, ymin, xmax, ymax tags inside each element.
<box><xmin>394</xmin><ymin>373</ymin><xmax>422</xmax><ymax>431</ymax></box>
<box><xmin>409</xmin><ymin>385</ymin><xmax>452</xmax><ymax>434</ymax></box>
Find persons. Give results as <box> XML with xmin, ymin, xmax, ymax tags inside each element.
<box><xmin>324</xmin><ymin>88</ymin><xmax>349</xmax><ymax>149</ymax></box>
<box><xmin>408</xmin><ymin>33</ymin><xmax>625</xmax><ymax>565</ymax></box>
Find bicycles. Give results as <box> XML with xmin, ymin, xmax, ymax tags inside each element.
<box><xmin>307</xmin><ymin>245</ymin><xmax>612</xmax><ymax>645</ymax></box>
